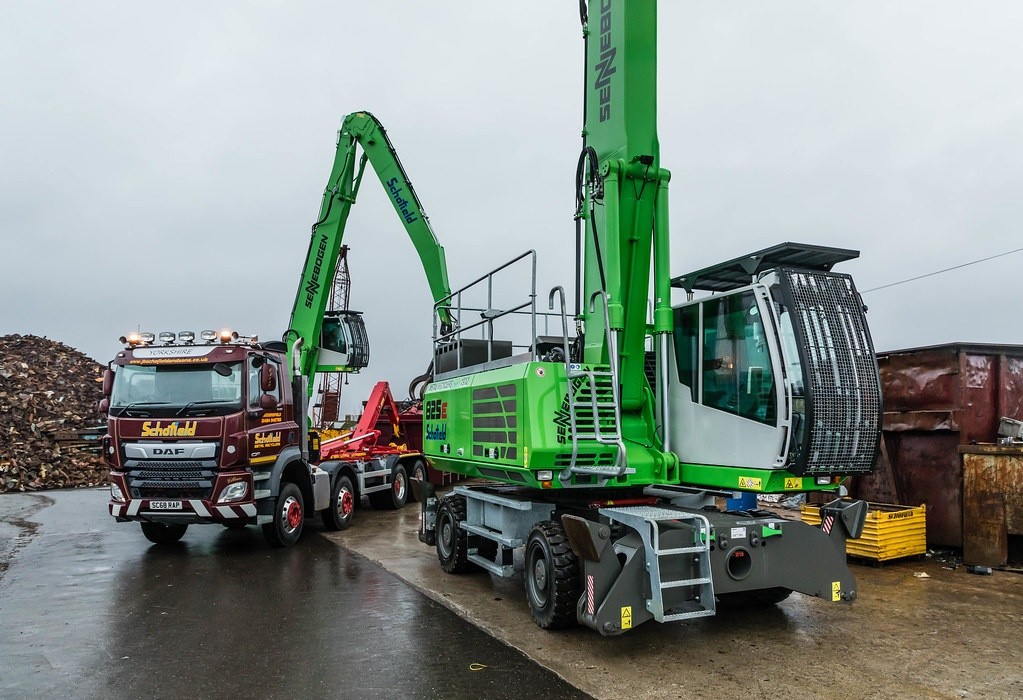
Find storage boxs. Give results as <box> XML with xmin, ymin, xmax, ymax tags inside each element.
<box><xmin>998</xmin><ymin>416</ymin><xmax>1023</xmax><ymax>437</ymax></box>
<box><xmin>800</xmin><ymin>501</ymin><xmax>927</xmax><ymax>562</ymax></box>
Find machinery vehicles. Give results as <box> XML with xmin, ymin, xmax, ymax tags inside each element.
<box><xmin>408</xmin><ymin>0</ymin><xmax>885</xmax><ymax>637</ymax></box>
<box><xmin>97</xmin><ymin>112</ymin><xmax>459</xmax><ymax>547</ymax></box>
<box><xmin>310</xmin><ymin>244</ymin><xmax>352</xmax><ymax>428</ymax></box>
